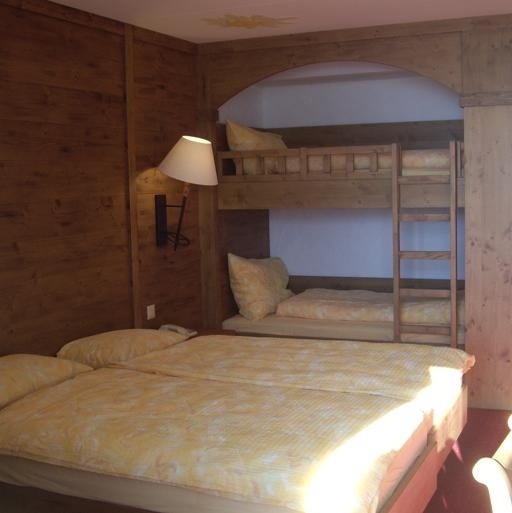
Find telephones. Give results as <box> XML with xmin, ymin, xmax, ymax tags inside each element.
<box><xmin>158</xmin><ymin>324</ymin><xmax>198</xmax><ymax>339</ymax></box>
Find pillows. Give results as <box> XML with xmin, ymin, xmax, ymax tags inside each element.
<box><xmin>58</xmin><ymin>328</ymin><xmax>191</xmax><ymax>368</ymax></box>
<box><xmin>1</xmin><ymin>355</ymin><xmax>93</xmax><ymax>409</ymax></box>
<box><xmin>225</xmin><ymin>119</ymin><xmax>289</xmax><ymax>175</ymax></box>
<box><xmin>228</xmin><ymin>250</ymin><xmax>297</xmax><ymax>322</ymax></box>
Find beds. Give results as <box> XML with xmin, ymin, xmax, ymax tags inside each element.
<box><xmin>1</xmin><ymin>354</ymin><xmax>439</xmax><ymax>513</ymax></box>
<box><xmin>216</xmin><ymin>120</ymin><xmax>466</xmax><ymax>349</ymax></box>
<box><xmin>57</xmin><ymin>328</ymin><xmax>477</xmax><ymax>476</ymax></box>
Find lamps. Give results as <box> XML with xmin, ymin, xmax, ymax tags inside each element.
<box><xmin>155</xmin><ymin>135</ymin><xmax>218</xmax><ymax>251</ymax></box>
